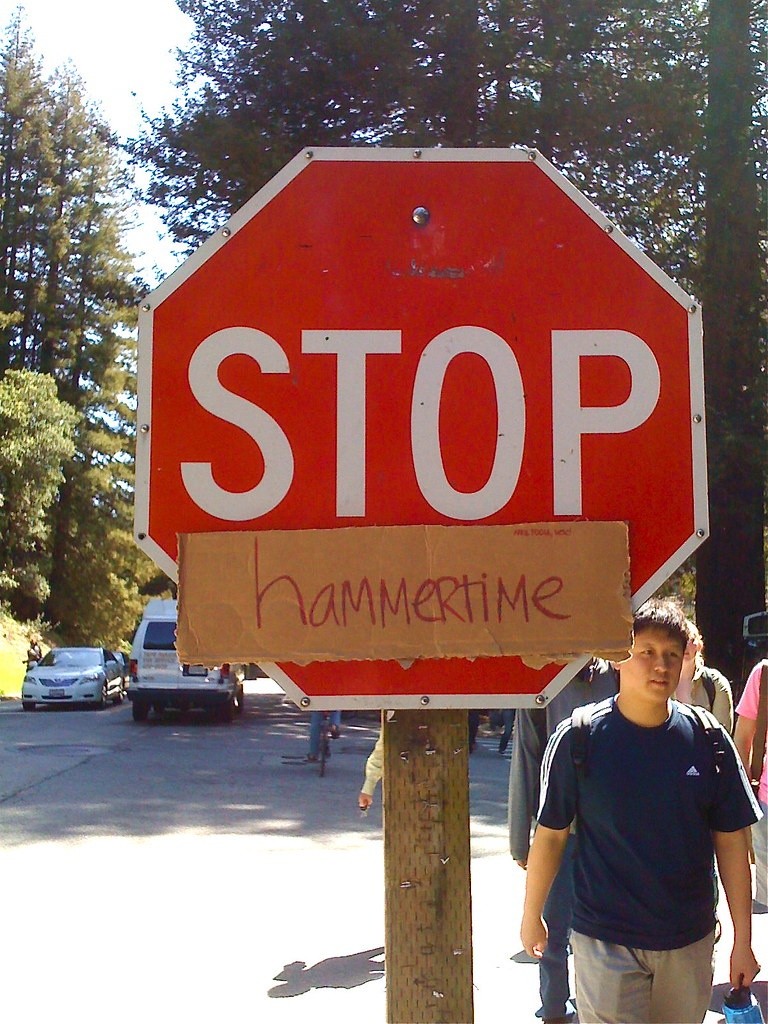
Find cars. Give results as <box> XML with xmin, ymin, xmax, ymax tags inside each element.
<box><xmin>22</xmin><ymin>646</ymin><xmax>129</xmax><ymax>711</ymax></box>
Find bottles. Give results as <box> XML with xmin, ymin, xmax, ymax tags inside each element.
<box><xmin>721</xmin><ymin>983</ymin><xmax>765</xmax><ymax>1024</ymax></box>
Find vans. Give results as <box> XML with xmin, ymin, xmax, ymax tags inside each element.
<box><xmin>126</xmin><ymin>598</ymin><xmax>246</xmax><ymax>723</ymax></box>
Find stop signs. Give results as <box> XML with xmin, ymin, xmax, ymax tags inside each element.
<box><xmin>132</xmin><ymin>146</ymin><xmax>709</xmax><ymax>710</ymax></box>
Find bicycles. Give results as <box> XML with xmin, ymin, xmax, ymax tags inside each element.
<box><xmin>308</xmin><ymin>712</ymin><xmax>340</xmax><ymax>776</ymax></box>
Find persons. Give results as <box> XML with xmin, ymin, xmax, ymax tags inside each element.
<box><xmin>359</xmin><ymin>737</ymin><xmax>382</xmax><ymax>809</ymax></box>
<box><xmin>22</xmin><ymin>634</ymin><xmax>42</xmax><ymax>670</ymax></box>
<box><xmin>308</xmin><ymin>710</ymin><xmax>341</xmax><ymax>762</ymax></box>
<box><xmin>670</xmin><ymin>621</ymin><xmax>733</xmax><ymax>735</ymax></box>
<box><xmin>521</xmin><ymin>600</ymin><xmax>761</xmax><ymax>1024</ymax></box>
<box><xmin>116</xmin><ymin>647</ymin><xmax>126</xmax><ymax>690</ymax></box>
<box><xmin>510</xmin><ymin>659</ymin><xmax>620</xmax><ymax>1024</ymax></box>
<box><xmin>732</xmin><ymin>660</ymin><xmax>767</xmax><ymax>914</ymax></box>
<box><xmin>468</xmin><ymin>708</ymin><xmax>514</xmax><ymax>754</ymax></box>
